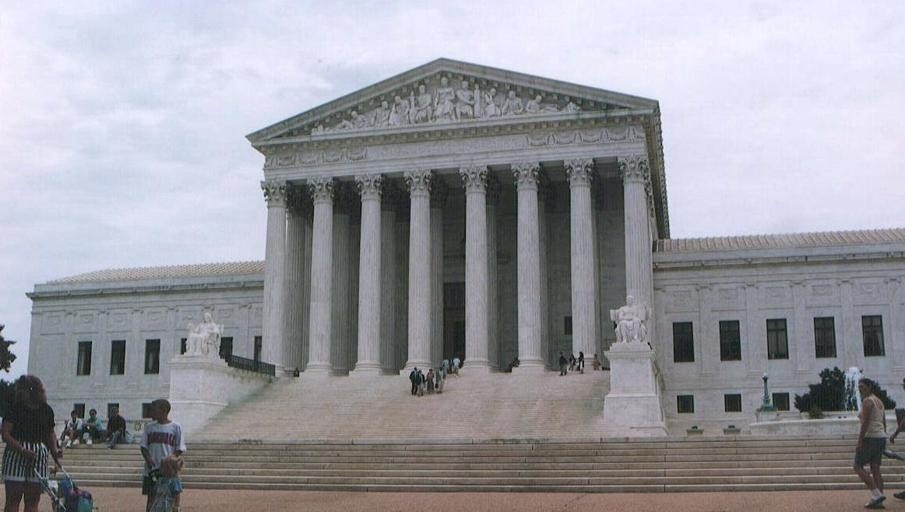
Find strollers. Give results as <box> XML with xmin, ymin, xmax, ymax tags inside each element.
<box><xmin>27</xmin><ymin>450</ymin><xmax>100</xmax><ymax>511</ymax></box>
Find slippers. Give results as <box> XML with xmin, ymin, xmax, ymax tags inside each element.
<box><xmin>864</xmin><ymin>496</ymin><xmax>886</xmax><ymax>509</ymax></box>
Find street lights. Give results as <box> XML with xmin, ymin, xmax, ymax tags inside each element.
<box><xmin>761</xmin><ymin>371</ymin><xmax>774</xmax><ymax>409</ymax></box>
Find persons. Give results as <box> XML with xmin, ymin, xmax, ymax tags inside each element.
<box><xmin>853</xmin><ymin>378</ymin><xmax>889</xmax><ymax>509</ymax></box>
<box><xmin>576</xmin><ymin>351</ymin><xmax>585</xmax><ymax>375</ymax></box>
<box><xmin>409</xmin><ymin>355</ymin><xmax>462</xmax><ymax>398</ymax></box>
<box><xmin>559</xmin><ymin>350</ymin><xmax>568</xmax><ymax>376</ymax></box>
<box><xmin>58</xmin><ymin>409</ymin><xmax>83</xmax><ymax>449</ymax></box>
<box><xmin>617</xmin><ymin>295</ymin><xmax>641</xmax><ymax>343</ymax></box>
<box><xmin>1</xmin><ymin>375</ymin><xmax>58</xmax><ymax>511</ymax></box>
<box><xmin>591</xmin><ymin>352</ymin><xmax>602</xmax><ymax>370</ymax></box>
<box><xmin>505</xmin><ymin>356</ymin><xmax>520</xmax><ymax>372</ymax></box>
<box><xmin>147</xmin><ymin>455</ymin><xmax>183</xmax><ymax>512</ymax></box>
<box><xmin>99</xmin><ymin>405</ymin><xmax>125</xmax><ymax>449</ymax></box>
<box><xmin>184</xmin><ymin>312</ymin><xmax>219</xmax><ymax>356</ymax></box>
<box><xmin>293</xmin><ymin>366</ymin><xmax>299</xmax><ymax>377</ymax></box>
<box><xmin>891</xmin><ymin>376</ymin><xmax>905</xmax><ymax>500</ymax></box>
<box><xmin>139</xmin><ymin>397</ymin><xmax>187</xmax><ymax>512</ymax></box>
<box><xmin>82</xmin><ymin>407</ymin><xmax>104</xmax><ymax>446</ymax></box>
<box><xmin>568</xmin><ymin>353</ymin><xmax>577</xmax><ymax>370</ymax></box>
